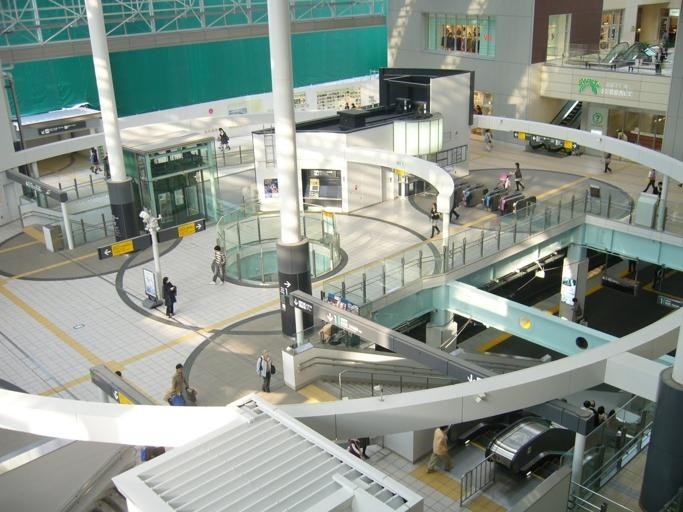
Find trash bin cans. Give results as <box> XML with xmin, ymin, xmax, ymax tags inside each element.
<box><xmin>42</xmin><ymin>224</ymin><xmax>64</xmax><ymax>253</ymax></box>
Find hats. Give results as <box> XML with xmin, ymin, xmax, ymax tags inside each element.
<box><xmin>176</xmin><ymin>364</ymin><xmax>184</xmax><ymax>369</ymax></box>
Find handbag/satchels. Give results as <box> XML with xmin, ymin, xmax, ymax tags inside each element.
<box><xmin>185</xmin><ymin>387</ymin><xmax>197</xmax><ymax>403</ymax></box>
<box><xmin>271</xmin><ymin>365</ymin><xmax>275</xmax><ymax>375</ymax></box>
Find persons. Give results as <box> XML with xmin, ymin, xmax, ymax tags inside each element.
<box><xmin>345</xmin><ymin>435</ymin><xmax>362</xmax><ymax>458</ymax></box>
<box><xmin>449</xmin><ymin>191</ymin><xmax>462</xmax><ymax>221</ymax></box>
<box><xmin>641</xmin><ymin>167</ymin><xmax>658</xmax><ymax>193</ymax></box>
<box><xmin>161</xmin><ymin>275</ymin><xmax>178</xmax><ymax>318</ymax></box>
<box><xmin>508</xmin><ymin>161</ymin><xmax>526</xmax><ymax>192</ymax></box>
<box><xmin>427</xmin><ymin>203</ymin><xmax>441</xmax><ymax>237</ymax></box>
<box><xmin>440</xmin><ymin>21</ymin><xmax>482</xmax><ymax>54</ymax></box>
<box><xmin>90</xmin><ymin>148</ymin><xmax>103</xmax><ymax>175</ymax></box>
<box><xmin>653</xmin><ymin>45</ymin><xmax>665</xmax><ymax>73</ymax></box>
<box><xmin>216</xmin><ymin>127</ymin><xmax>231</xmax><ymax>149</ymax></box>
<box><xmin>484</xmin><ymin>128</ymin><xmax>493</xmax><ymax>150</ymax></box>
<box><xmin>580</xmin><ymin>398</ymin><xmax>618</xmax><ymax>424</ymax></box>
<box><xmin>208</xmin><ymin>244</ymin><xmax>227</xmax><ymax>285</ymax></box>
<box><xmin>653</xmin><ymin>181</ymin><xmax>665</xmax><ymax>195</ymax></box>
<box><xmin>168</xmin><ymin>363</ymin><xmax>191</xmax><ymax>405</ymax></box>
<box><xmin>426</xmin><ymin>423</ymin><xmax>454</xmax><ymax>473</ymax></box>
<box><xmin>256</xmin><ymin>348</ymin><xmax>277</xmax><ymax>393</ymax></box>
<box><xmin>602</xmin><ymin>151</ymin><xmax>612</xmax><ymax>173</ymax></box>
<box><xmin>569</xmin><ymin>297</ymin><xmax>585</xmax><ymax>320</ymax></box>
<box><xmin>614</xmin><ymin>128</ymin><xmax>627</xmax><ymax>141</ymax></box>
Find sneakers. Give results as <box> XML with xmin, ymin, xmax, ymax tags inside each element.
<box><xmin>209</xmin><ymin>280</ymin><xmax>223</xmax><ymax>285</ymax></box>
